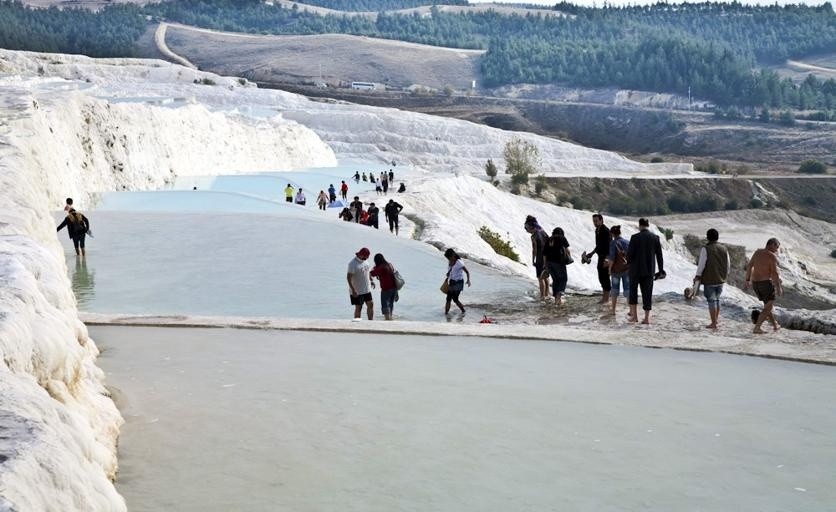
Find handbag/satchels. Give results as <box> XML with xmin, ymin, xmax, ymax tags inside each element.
<box><xmin>440</xmin><ymin>277</ymin><xmax>449</xmax><ymax>293</ymax></box>
<box><xmin>563</xmin><ymin>246</ymin><xmax>574</xmax><ymax>265</ymax></box>
<box><xmin>611</xmin><ymin>252</ymin><xmax>627</xmax><ymax>272</ymax></box>
<box><xmin>393</xmin><ymin>269</ymin><xmax>405</xmax><ymax>289</ymax></box>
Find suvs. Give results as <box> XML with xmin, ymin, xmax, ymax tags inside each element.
<box><xmin>317</xmin><ymin>83</ymin><xmax>327</xmax><ymax>88</ymax></box>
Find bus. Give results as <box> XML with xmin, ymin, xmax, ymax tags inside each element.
<box><xmin>351</xmin><ymin>80</ymin><xmax>376</xmax><ymax>91</ymax></box>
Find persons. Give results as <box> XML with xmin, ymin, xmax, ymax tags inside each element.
<box><xmin>315</xmin><ymin>169</ymin><xmax>406</xmax><ymax>237</ymax></box>
<box><xmin>443</xmin><ymin>248</ymin><xmax>470</xmax><ymax>314</ymax></box>
<box><xmin>523</xmin><ymin>212</ymin><xmax>663</xmax><ymax>324</ymax></box>
<box><xmin>283</xmin><ymin>183</ymin><xmax>294</xmax><ymax>203</ymax></box>
<box><xmin>346</xmin><ymin>248</ymin><xmax>374</xmax><ymax>320</ymax></box>
<box><xmin>692</xmin><ymin>228</ymin><xmax>731</xmax><ymax>328</ymax></box>
<box><xmin>369</xmin><ymin>253</ymin><xmax>397</xmax><ymax>321</ymax></box>
<box><xmin>56</xmin><ymin>207</ymin><xmax>90</xmax><ymax>257</ymax></box>
<box><xmin>295</xmin><ymin>188</ymin><xmax>305</xmax><ymax>205</ymax></box>
<box><xmin>64</xmin><ymin>197</ymin><xmax>74</xmax><ymax>212</ymax></box>
<box><xmin>744</xmin><ymin>237</ymin><xmax>783</xmax><ymax>333</ymax></box>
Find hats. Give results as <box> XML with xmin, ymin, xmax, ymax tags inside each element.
<box><xmin>356</xmin><ymin>248</ymin><xmax>370</xmax><ymax>256</ymax></box>
